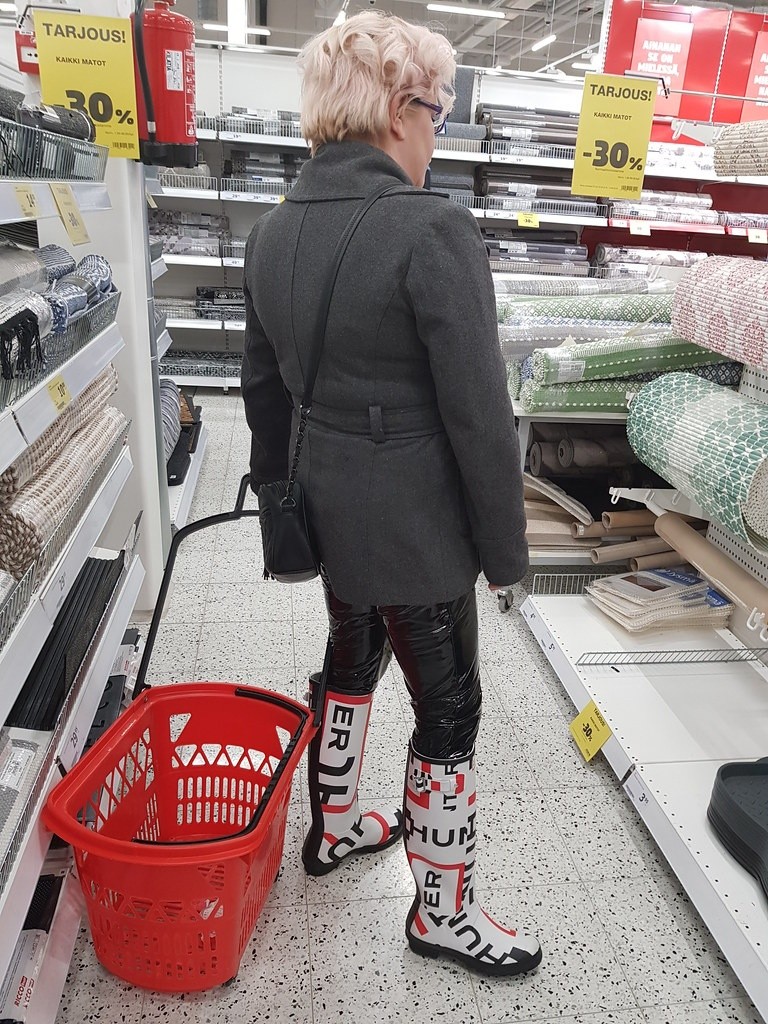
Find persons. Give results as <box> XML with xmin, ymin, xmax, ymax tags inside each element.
<box><xmin>241</xmin><ymin>12</ymin><xmax>542</xmax><ymax>978</ymax></box>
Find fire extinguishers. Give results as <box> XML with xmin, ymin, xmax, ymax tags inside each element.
<box><xmin>127</xmin><ymin>1</ymin><xmax>194</xmax><ymax>142</ymax></box>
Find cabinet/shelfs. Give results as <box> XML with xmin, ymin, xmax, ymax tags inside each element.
<box><xmin>0</xmin><ymin>2</ymin><xmax>768</xmax><ymax>1024</ymax></box>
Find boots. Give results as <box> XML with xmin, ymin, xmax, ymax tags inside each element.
<box><xmin>403</xmin><ymin>738</ymin><xmax>542</xmax><ymax>975</ymax></box>
<box><xmin>302</xmin><ymin>670</ymin><xmax>403</xmax><ymax>876</ymax></box>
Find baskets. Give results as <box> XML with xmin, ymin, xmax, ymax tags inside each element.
<box><xmin>44</xmin><ymin>682</ymin><xmax>321</xmax><ymax>992</ymax></box>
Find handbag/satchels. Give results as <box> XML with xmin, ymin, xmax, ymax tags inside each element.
<box><xmin>258</xmin><ymin>479</ymin><xmax>320</xmax><ymax>583</ymax></box>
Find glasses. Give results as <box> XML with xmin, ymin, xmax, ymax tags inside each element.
<box><xmin>415</xmin><ymin>98</ymin><xmax>450</xmax><ymax>134</ymax></box>
